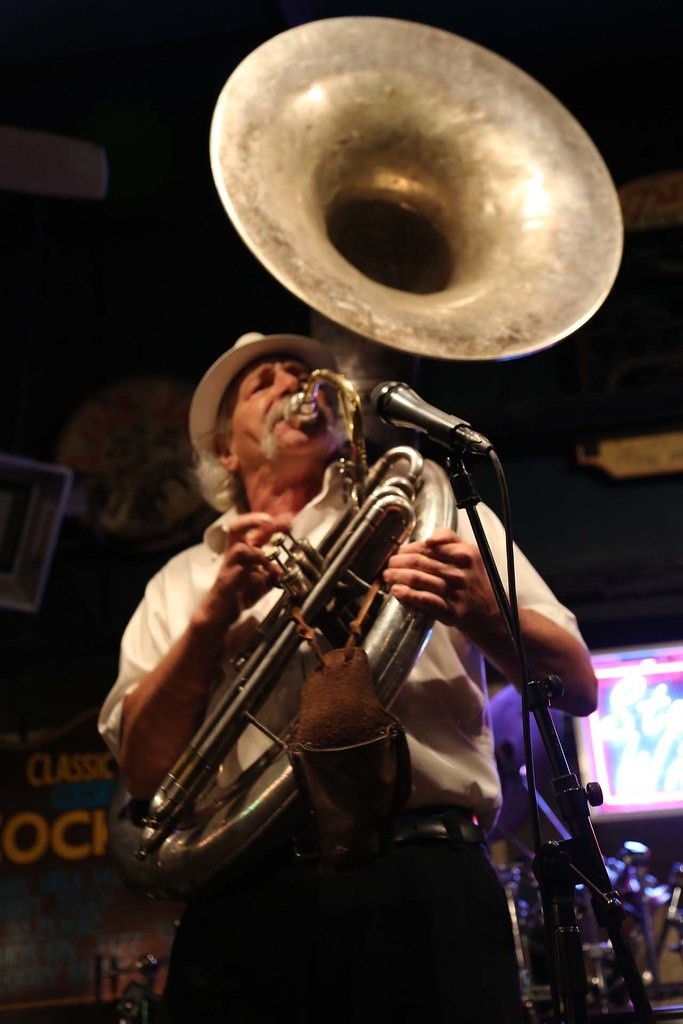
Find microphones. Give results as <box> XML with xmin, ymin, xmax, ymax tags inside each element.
<box><xmin>371</xmin><ymin>381</ymin><xmax>495</xmax><ymax>456</ymax></box>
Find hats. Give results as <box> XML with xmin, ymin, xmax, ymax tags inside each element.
<box><xmin>188</xmin><ymin>331</ymin><xmax>340</xmax><ymax>466</ymax></box>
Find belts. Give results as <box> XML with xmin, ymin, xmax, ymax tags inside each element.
<box><xmin>231</xmin><ymin>817</ymin><xmax>480</xmax><ymax>869</ymax></box>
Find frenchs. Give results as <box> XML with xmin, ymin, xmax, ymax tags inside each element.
<box><xmin>131</xmin><ymin>12</ymin><xmax>635</xmax><ymax>895</ymax></box>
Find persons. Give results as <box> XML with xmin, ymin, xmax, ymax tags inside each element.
<box><xmin>96</xmin><ymin>331</ymin><xmax>598</xmax><ymax>1022</ymax></box>
